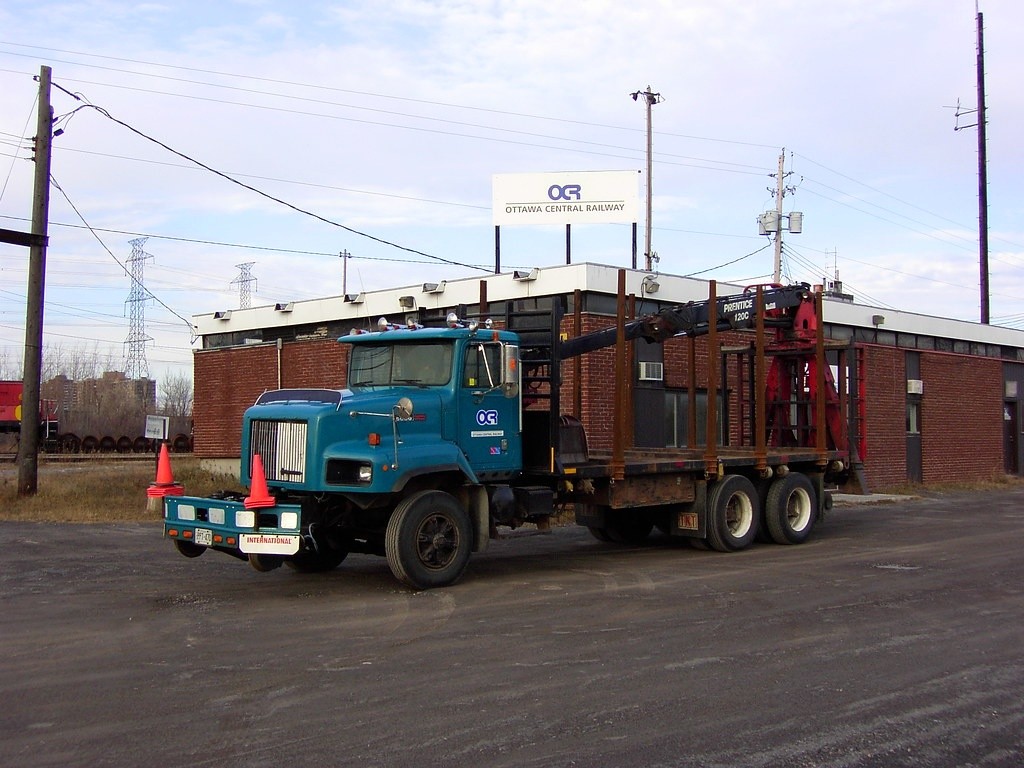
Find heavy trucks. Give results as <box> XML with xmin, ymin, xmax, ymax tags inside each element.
<box><xmin>162</xmin><ymin>268</ymin><xmax>870</xmax><ymax>592</ymax></box>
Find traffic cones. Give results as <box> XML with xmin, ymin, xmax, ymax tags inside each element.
<box><xmin>147</xmin><ymin>442</ymin><xmax>184</xmax><ymax>497</ymax></box>
<box><xmin>245</xmin><ymin>454</ymin><xmax>276</xmax><ymax>509</ymax></box>
